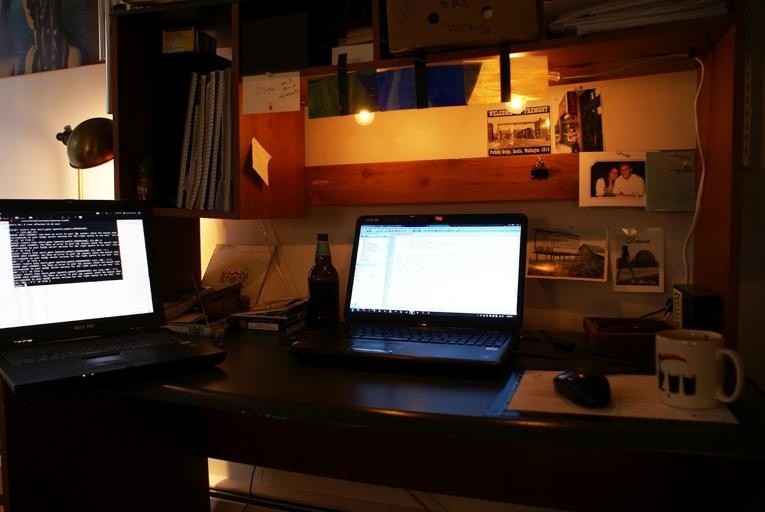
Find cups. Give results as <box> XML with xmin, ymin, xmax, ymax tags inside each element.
<box><xmin>654</xmin><ymin>328</ymin><xmax>743</xmax><ymax>411</ymax></box>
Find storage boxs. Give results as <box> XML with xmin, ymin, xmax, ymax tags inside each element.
<box><xmin>384</xmin><ymin>0</ymin><xmax>539</xmax><ymax>57</ymax></box>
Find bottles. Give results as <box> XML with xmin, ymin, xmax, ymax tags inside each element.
<box><xmin>308</xmin><ymin>232</ymin><xmax>338</xmax><ymax>327</ymax></box>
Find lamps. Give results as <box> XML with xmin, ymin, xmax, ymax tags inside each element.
<box><xmin>58</xmin><ymin>118</ymin><xmax>114</xmax><ymax>170</ymax></box>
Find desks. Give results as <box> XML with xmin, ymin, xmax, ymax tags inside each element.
<box><xmin>156</xmin><ymin>291</ymin><xmax>763</xmax><ymax>512</ymax></box>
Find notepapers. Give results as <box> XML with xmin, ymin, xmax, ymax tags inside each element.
<box><xmin>251</xmin><ymin>136</ymin><xmax>273</xmax><ymax>188</ymax></box>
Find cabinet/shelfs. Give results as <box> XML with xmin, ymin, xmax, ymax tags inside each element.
<box><xmin>106</xmin><ymin>0</ymin><xmax>738</xmax><ymax>363</ymax></box>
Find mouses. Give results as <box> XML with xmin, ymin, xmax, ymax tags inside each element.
<box><xmin>552</xmin><ymin>367</ymin><xmax>612</xmax><ymax>408</ymax></box>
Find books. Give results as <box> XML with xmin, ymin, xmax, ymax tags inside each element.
<box><xmin>331</xmin><ymin>26</ymin><xmax>375</xmax><ymax>64</ymax></box>
<box><xmin>178</xmin><ymin>67</ymin><xmax>233</xmax><ymax>211</ymax></box>
<box><xmin>549</xmin><ymin>0</ymin><xmax>730</xmax><ymax>34</ymax></box>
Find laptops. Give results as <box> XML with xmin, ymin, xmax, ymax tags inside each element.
<box><xmin>337</xmin><ymin>213</ymin><xmax>529</xmax><ymax>365</ymax></box>
<box><xmin>1</xmin><ymin>199</ymin><xmax>227</xmax><ymax>395</ymax></box>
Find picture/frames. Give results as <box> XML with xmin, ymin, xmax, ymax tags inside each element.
<box><xmin>577</xmin><ymin>150</ymin><xmax>648</xmax><ymax>206</ymax></box>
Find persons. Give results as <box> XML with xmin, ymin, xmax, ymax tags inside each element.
<box><xmin>613</xmin><ymin>165</ymin><xmax>644</xmax><ymax>196</ymax></box>
<box><xmin>595</xmin><ymin>168</ymin><xmax>619</xmax><ymax>197</ymax></box>
